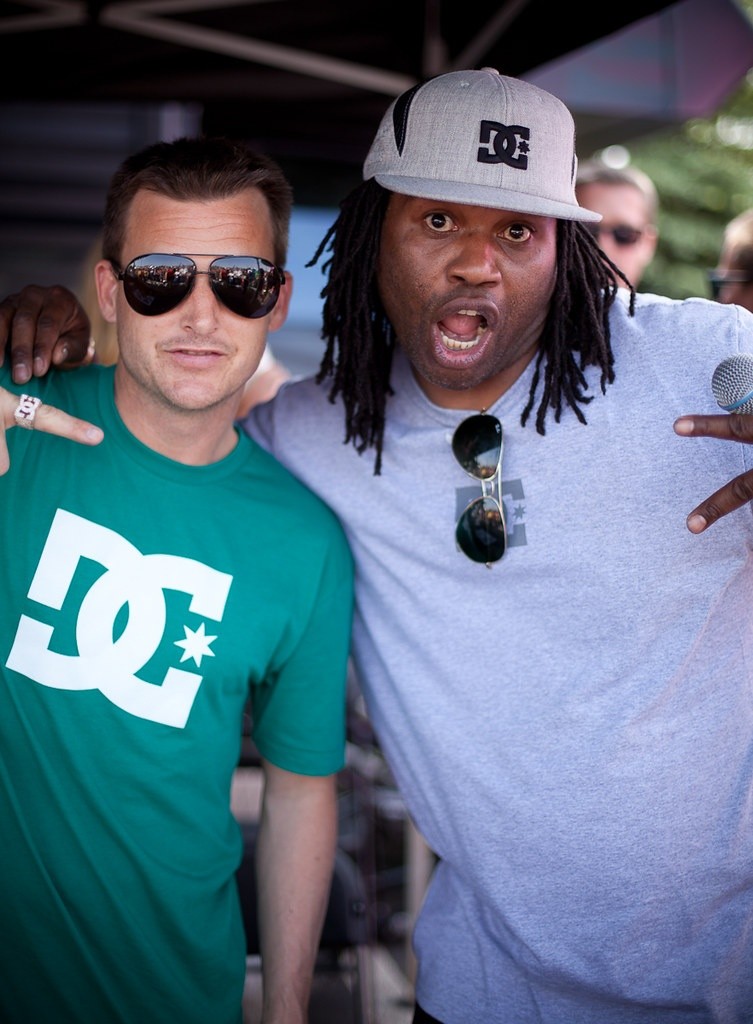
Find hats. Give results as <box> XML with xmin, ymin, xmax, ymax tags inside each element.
<box><xmin>363</xmin><ymin>67</ymin><xmax>603</xmax><ymax>222</ymax></box>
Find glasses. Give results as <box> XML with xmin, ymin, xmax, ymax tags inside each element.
<box><xmin>706</xmin><ymin>273</ymin><xmax>752</xmax><ymax>298</ymax></box>
<box><xmin>451</xmin><ymin>407</ymin><xmax>506</xmax><ymax>569</ymax></box>
<box><xmin>111</xmin><ymin>253</ymin><xmax>285</xmax><ymax>319</ymax></box>
<box><xmin>586</xmin><ymin>223</ymin><xmax>644</xmax><ymax>244</ymax></box>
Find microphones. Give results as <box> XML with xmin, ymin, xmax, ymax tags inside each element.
<box><xmin>711</xmin><ymin>353</ymin><xmax>753</xmax><ymax>414</ymax></box>
